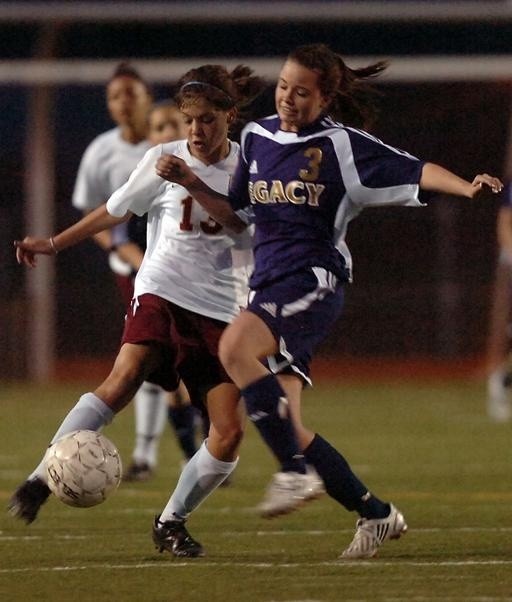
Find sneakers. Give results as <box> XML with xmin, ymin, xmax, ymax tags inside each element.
<box><xmin>129</xmin><ymin>460</ymin><xmax>150</xmax><ymax>479</ymax></box>
<box><xmin>152</xmin><ymin>515</ymin><xmax>204</xmax><ymax>558</ymax></box>
<box><xmin>255</xmin><ymin>465</ymin><xmax>327</xmax><ymax>516</ymax></box>
<box><xmin>8</xmin><ymin>479</ymin><xmax>50</xmax><ymax>524</ymax></box>
<box><xmin>339</xmin><ymin>501</ymin><xmax>408</xmax><ymax>559</ymax></box>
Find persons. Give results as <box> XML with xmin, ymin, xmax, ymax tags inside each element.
<box><xmin>155</xmin><ymin>49</ymin><xmax>504</xmax><ymax>559</ymax></box>
<box><xmin>486</xmin><ymin>139</ymin><xmax>512</xmax><ymax>423</ymax></box>
<box><xmin>110</xmin><ymin>104</ymin><xmax>236</xmax><ymax>485</ymax></box>
<box><xmin>68</xmin><ymin>57</ymin><xmax>205</xmax><ymax>482</ymax></box>
<box><xmin>10</xmin><ymin>62</ymin><xmax>258</xmax><ymax>559</ymax></box>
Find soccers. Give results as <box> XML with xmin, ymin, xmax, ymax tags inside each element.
<box><xmin>45</xmin><ymin>430</ymin><xmax>122</xmax><ymax>508</ymax></box>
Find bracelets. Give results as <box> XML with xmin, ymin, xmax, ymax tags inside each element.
<box><xmin>50</xmin><ymin>237</ymin><xmax>57</xmax><ymax>253</ymax></box>
